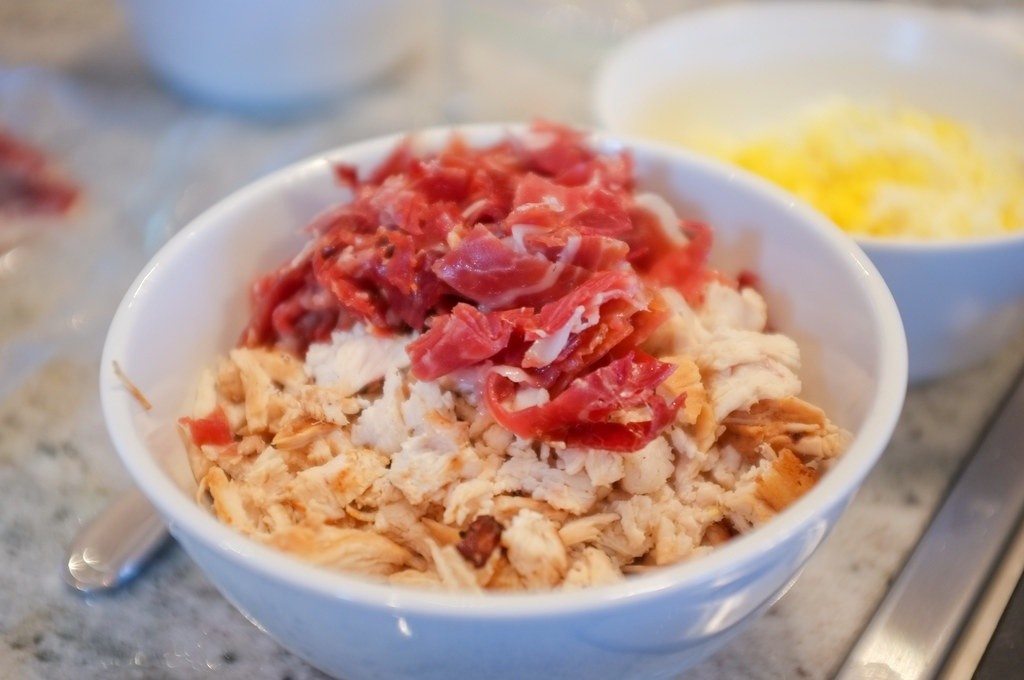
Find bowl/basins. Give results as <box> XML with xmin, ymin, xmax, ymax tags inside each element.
<box><xmin>592</xmin><ymin>0</ymin><xmax>1024</xmax><ymax>379</ymax></box>
<box><xmin>100</xmin><ymin>123</ymin><xmax>909</xmax><ymax>680</ymax></box>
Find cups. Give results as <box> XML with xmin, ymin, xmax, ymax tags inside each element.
<box><xmin>133</xmin><ymin>1</ymin><xmax>436</xmax><ymax>115</ymax></box>
